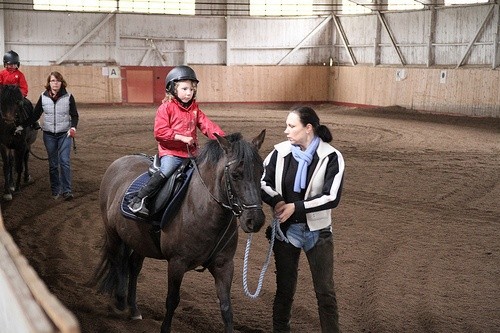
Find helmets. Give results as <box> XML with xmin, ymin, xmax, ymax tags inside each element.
<box><xmin>3</xmin><ymin>50</ymin><xmax>20</xmax><ymax>69</ymax></box>
<box><xmin>165</xmin><ymin>65</ymin><xmax>200</xmax><ymax>88</ymax></box>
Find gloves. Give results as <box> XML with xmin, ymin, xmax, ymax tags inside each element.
<box><xmin>67</xmin><ymin>127</ymin><xmax>76</xmax><ymax>139</ymax></box>
<box><xmin>13</xmin><ymin>125</ymin><xmax>24</xmax><ymax>136</ymax></box>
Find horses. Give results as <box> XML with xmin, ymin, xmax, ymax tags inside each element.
<box><xmin>0</xmin><ymin>84</ymin><xmax>38</xmax><ymax>202</ymax></box>
<box><xmin>91</xmin><ymin>129</ymin><xmax>266</xmax><ymax>333</ymax></box>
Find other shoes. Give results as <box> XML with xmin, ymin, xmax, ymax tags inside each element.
<box><xmin>50</xmin><ymin>192</ymin><xmax>59</xmax><ymax>200</ymax></box>
<box><xmin>63</xmin><ymin>192</ymin><xmax>74</xmax><ymax>201</ymax></box>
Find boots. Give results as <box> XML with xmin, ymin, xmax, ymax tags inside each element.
<box><xmin>126</xmin><ymin>172</ymin><xmax>166</xmax><ymax>217</ymax></box>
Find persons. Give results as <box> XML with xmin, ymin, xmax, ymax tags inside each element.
<box><xmin>260</xmin><ymin>105</ymin><xmax>345</xmax><ymax>333</ymax></box>
<box><xmin>0</xmin><ymin>50</ymin><xmax>41</xmax><ymax>128</ymax></box>
<box><xmin>129</xmin><ymin>66</ymin><xmax>226</xmax><ymax>216</ymax></box>
<box><xmin>13</xmin><ymin>71</ymin><xmax>79</xmax><ymax>200</ymax></box>
